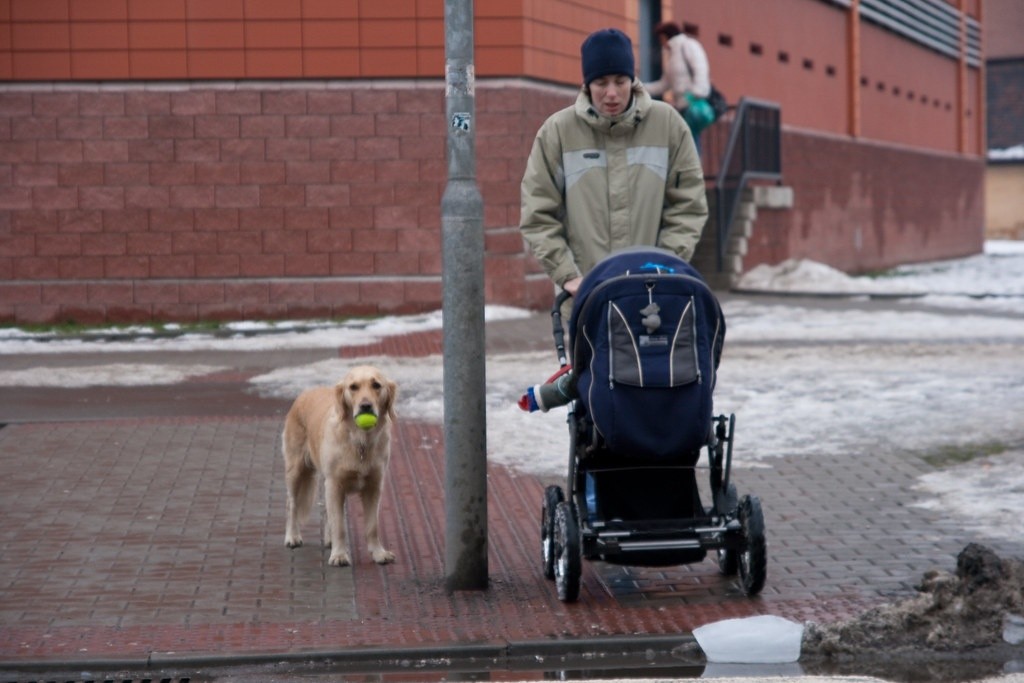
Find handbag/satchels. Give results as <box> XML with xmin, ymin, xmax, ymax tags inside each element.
<box><xmin>678</xmin><ymin>88</ymin><xmax>714</xmax><ymax>131</ymax></box>
<box><xmin>705</xmin><ymin>87</ymin><xmax>727</xmax><ymax>119</ymax></box>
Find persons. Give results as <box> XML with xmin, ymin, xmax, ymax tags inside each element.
<box><xmin>516</xmin><ymin>369</ymin><xmax>579</xmax><ymax>414</ymax></box>
<box><xmin>641</xmin><ymin>21</ymin><xmax>711</xmax><ymax>156</ymax></box>
<box><xmin>519</xmin><ymin>27</ymin><xmax>708</xmax><ymax>340</ymax></box>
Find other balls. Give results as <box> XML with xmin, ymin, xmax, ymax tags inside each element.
<box><xmin>356</xmin><ymin>413</ymin><xmax>376</xmax><ymax>430</ymax></box>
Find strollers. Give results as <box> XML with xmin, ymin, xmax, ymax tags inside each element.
<box><xmin>539</xmin><ymin>247</ymin><xmax>769</xmax><ymax>605</ymax></box>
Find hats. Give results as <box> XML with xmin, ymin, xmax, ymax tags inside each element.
<box><xmin>579</xmin><ymin>27</ymin><xmax>635</xmax><ymax>88</ymax></box>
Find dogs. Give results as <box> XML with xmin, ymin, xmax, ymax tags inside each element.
<box><xmin>275</xmin><ymin>364</ymin><xmax>402</xmax><ymax>571</ymax></box>
